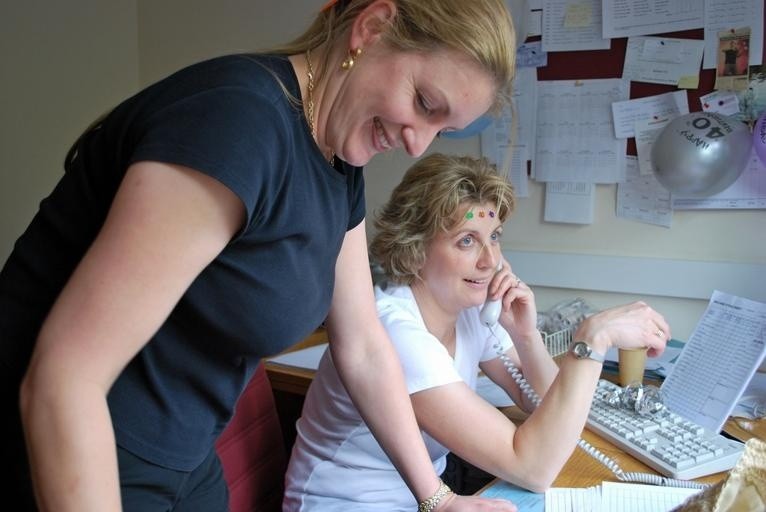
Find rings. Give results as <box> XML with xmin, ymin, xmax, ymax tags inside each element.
<box><xmin>656</xmin><ymin>328</ymin><xmax>666</xmax><ymax>340</ymax></box>
<box><xmin>516</xmin><ymin>281</ymin><xmax>521</xmax><ymax>288</ymax></box>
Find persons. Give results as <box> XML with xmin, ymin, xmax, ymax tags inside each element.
<box><xmin>0</xmin><ymin>0</ymin><xmax>519</xmax><ymax>511</ymax></box>
<box><xmin>281</xmin><ymin>152</ymin><xmax>673</xmax><ymax>512</ymax></box>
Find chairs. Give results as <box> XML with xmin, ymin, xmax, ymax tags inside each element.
<box><xmin>215</xmin><ymin>362</ymin><xmax>289</xmax><ymax>512</ymax></box>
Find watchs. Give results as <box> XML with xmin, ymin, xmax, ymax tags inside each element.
<box><xmin>568</xmin><ymin>340</ymin><xmax>605</xmax><ymax>363</ymax></box>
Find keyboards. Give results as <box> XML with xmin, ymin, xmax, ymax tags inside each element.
<box><xmin>584</xmin><ymin>379</ymin><xmax>746</xmax><ymax>480</ymax></box>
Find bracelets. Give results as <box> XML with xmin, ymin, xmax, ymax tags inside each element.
<box><xmin>418</xmin><ymin>483</ymin><xmax>452</xmax><ymax>511</ymax></box>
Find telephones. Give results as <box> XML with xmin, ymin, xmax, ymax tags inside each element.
<box><xmin>479</xmin><ymin>263</ymin><xmax>506</xmax><ymax>329</ymax></box>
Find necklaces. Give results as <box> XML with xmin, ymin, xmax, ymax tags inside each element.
<box><xmin>304</xmin><ymin>49</ymin><xmax>337</xmax><ymax>169</ymax></box>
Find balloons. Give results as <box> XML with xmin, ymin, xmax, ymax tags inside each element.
<box><xmin>434</xmin><ymin>113</ymin><xmax>493</xmax><ymax>139</ymax></box>
<box><xmin>753</xmin><ymin>113</ymin><xmax>766</xmax><ymax>168</ymax></box>
<box><xmin>650</xmin><ymin>111</ymin><xmax>753</xmax><ymax>200</ymax></box>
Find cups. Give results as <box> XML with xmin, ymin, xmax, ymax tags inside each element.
<box><xmin>618</xmin><ymin>346</ymin><xmax>650</xmax><ymax>389</ymax></box>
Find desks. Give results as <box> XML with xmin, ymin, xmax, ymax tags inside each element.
<box><xmin>264</xmin><ymin>329</ymin><xmax>616</xmax><ymax>427</ymax></box>
<box><xmin>469</xmin><ymin>378</ymin><xmax>766</xmax><ymax>511</ymax></box>
